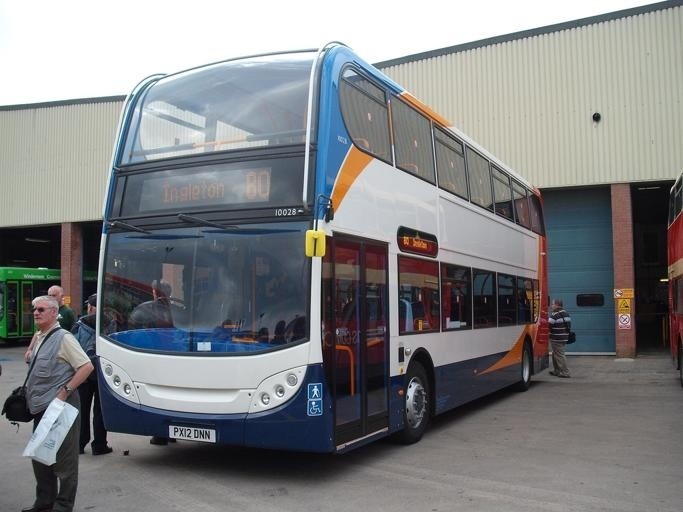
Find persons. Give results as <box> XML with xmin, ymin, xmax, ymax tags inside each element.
<box><xmin>22</xmin><ymin>295</ymin><xmax>94</xmax><ymax>512</ymax></box>
<box><xmin>70</xmin><ymin>293</ymin><xmax>113</xmax><ymax>455</ymax></box>
<box><xmin>127</xmin><ymin>279</ymin><xmax>188</xmax><ymax>330</ymax></box>
<box><xmin>549</xmin><ymin>297</ymin><xmax>572</xmax><ymax>377</ymax></box>
<box><xmin>47</xmin><ymin>285</ymin><xmax>78</xmax><ymax>331</ymax></box>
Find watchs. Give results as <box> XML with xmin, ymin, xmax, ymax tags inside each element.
<box><xmin>63</xmin><ymin>384</ymin><xmax>73</xmax><ymax>396</ymax></box>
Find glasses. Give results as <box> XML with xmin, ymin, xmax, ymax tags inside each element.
<box><xmin>31</xmin><ymin>307</ymin><xmax>51</xmax><ymax>313</ymax></box>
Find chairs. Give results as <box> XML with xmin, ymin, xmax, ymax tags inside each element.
<box><xmin>352</xmin><ymin>138</ymin><xmax>529</xmax><ymax>224</ymax></box>
<box><xmin>415</xmin><ymin>316</ymin><xmax>527</xmax><ymax>332</ymax></box>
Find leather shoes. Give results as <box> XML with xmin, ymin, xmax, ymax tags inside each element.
<box><xmin>79</xmin><ymin>447</ymin><xmax>113</xmax><ymax>455</ymax></box>
<box><xmin>150</xmin><ymin>437</ymin><xmax>177</xmax><ymax>444</ymax></box>
<box><xmin>21</xmin><ymin>506</ymin><xmax>50</xmax><ymax>512</ymax></box>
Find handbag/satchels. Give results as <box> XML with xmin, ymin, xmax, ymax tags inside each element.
<box><xmin>566</xmin><ymin>332</ymin><xmax>576</xmax><ymax>344</ymax></box>
<box><xmin>1</xmin><ymin>386</ymin><xmax>33</xmax><ymax>433</ymax></box>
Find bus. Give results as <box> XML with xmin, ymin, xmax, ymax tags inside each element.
<box><xmin>89</xmin><ymin>39</ymin><xmax>549</xmax><ymax>458</ymax></box>
<box><xmin>666</xmin><ymin>170</ymin><xmax>683</xmax><ymax>386</ymax></box>
<box><xmin>0</xmin><ymin>266</ymin><xmax>98</xmax><ymax>346</ymax></box>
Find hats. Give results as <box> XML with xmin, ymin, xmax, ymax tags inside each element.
<box><xmin>151</xmin><ymin>280</ymin><xmax>172</xmax><ymax>296</ymax></box>
<box><xmin>84</xmin><ymin>293</ymin><xmax>97</xmax><ymax>307</ymax></box>
